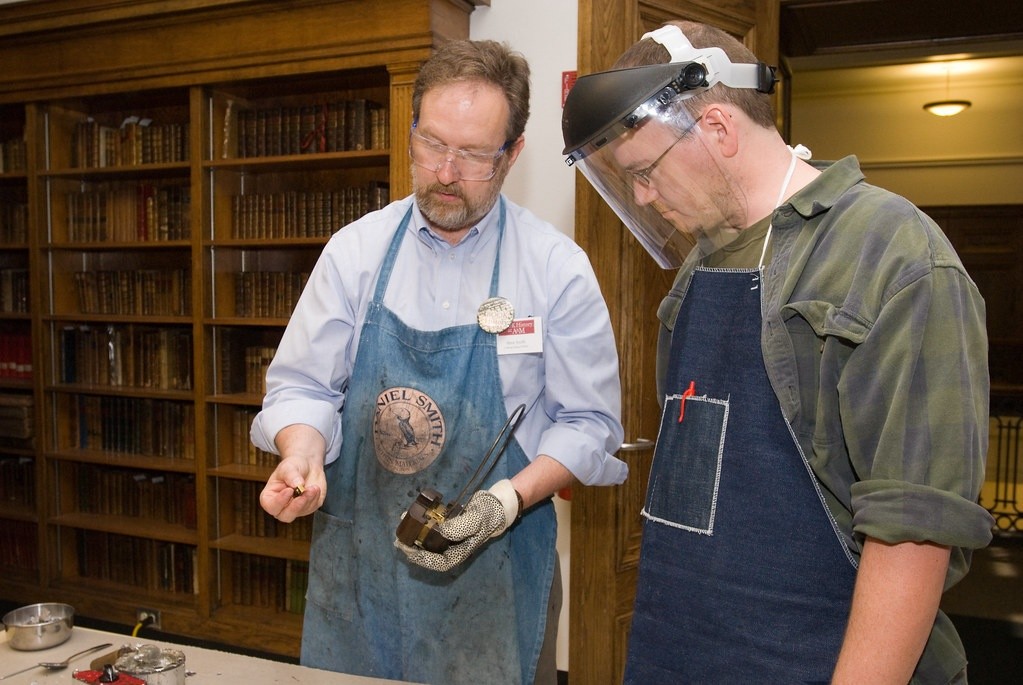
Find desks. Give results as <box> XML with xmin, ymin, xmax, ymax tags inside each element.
<box><xmin>0</xmin><ymin>624</ymin><xmax>417</xmax><ymax>685</ymax></box>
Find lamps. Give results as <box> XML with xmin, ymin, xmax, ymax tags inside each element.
<box><xmin>923</xmin><ymin>65</ymin><xmax>973</xmax><ymax>119</ymax></box>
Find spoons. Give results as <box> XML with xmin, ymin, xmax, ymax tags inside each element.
<box><xmin>39</xmin><ymin>643</ymin><xmax>112</xmax><ymax>670</ymax></box>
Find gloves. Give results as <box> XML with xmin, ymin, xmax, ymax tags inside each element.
<box><xmin>394</xmin><ymin>479</ymin><xmax>520</xmax><ymax>572</ymax></box>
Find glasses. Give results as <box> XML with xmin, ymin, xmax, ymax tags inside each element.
<box><xmin>408</xmin><ymin>123</ymin><xmax>514</xmax><ymax>181</ymax></box>
<box><xmin>623</xmin><ymin>115</ymin><xmax>704</xmax><ymax>186</ymax></box>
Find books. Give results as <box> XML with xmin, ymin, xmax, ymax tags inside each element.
<box><xmin>221</xmin><ymin>97</ymin><xmax>390</xmax><ymax>615</ymax></box>
<box><xmin>59</xmin><ymin>120</ymin><xmax>199</xmax><ymax>597</ymax></box>
<box><xmin>0</xmin><ymin>139</ymin><xmax>39</xmax><ymax>572</ymax></box>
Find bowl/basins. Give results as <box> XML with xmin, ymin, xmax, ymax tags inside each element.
<box><xmin>2</xmin><ymin>602</ymin><xmax>75</xmax><ymax>651</ymax></box>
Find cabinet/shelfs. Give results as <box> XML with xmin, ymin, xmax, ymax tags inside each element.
<box><xmin>0</xmin><ymin>0</ymin><xmax>488</xmax><ymax>659</ymax></box>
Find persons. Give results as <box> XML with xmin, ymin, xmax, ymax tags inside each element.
<box><xmin>250</xmin><ymin>41</ymin><xmax>628</xmax><ymax>685</ymax></box>
<box><xmin>562</xmin><ymin>21</ymin><xmax>993</xmax><ymax>685</ymax></box>
